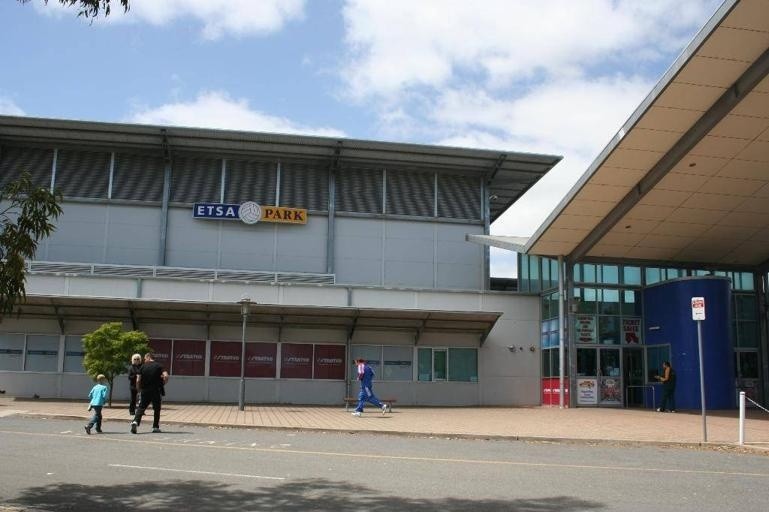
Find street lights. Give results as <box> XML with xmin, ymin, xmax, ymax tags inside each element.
<box><xmin>236</xmin><ymin>298</ymin><xmax>257</xmax><ymax>411</ymax></box>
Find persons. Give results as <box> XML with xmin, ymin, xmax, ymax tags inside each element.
<box><xmin>130</xmin><ymin>352</ymin><xmax>169</xmax><ymax>434</ymax></box>
<box><xmin>85</xmin><ymin>373</ymin><xmax>108</xmax><ymax>434</ymax></box>
<box><xmin>352</xmin><ymin>358</ymin><xmax>387</xmax><ymax>416</ymax></box>
<box><xmin>653</xmin><ymin>361</ymin><xmax>676</xmax><ymax>412</ymax></box>
<box><xmin>128</xmin><ymin>353</ymin><xmax>147</xmax><ymax>415</ymax></box>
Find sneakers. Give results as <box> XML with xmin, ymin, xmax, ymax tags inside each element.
<box><xmin>84</xmin><ymin>425</ymin><xmax>102</xmax><ymax>434</ymax></box>
<box><xmin>131</xmin><ymin>421</ymin><xmax>161</xmax><ymax>433</ymax></box>
<box><xmin>352</xmin><ymin>411</ymin><xmax>361</xmax><ymax>417</ymax></box>
<box><xmin>381</xmin><ymin>405</ymin><xmax>387</xmax><ymax>415</ymax></box>
